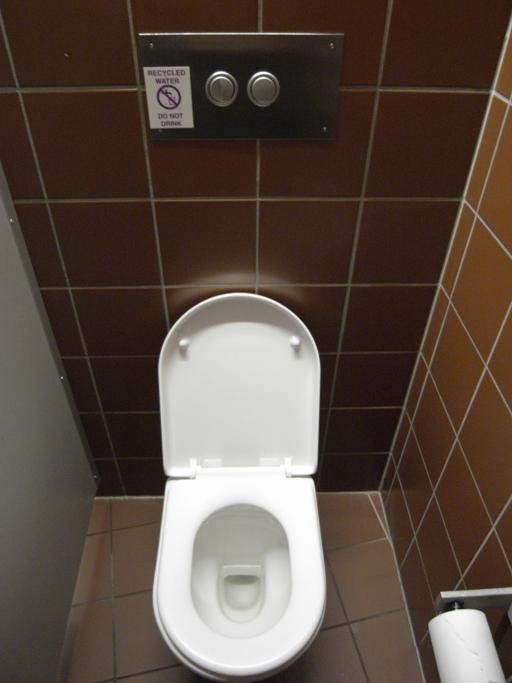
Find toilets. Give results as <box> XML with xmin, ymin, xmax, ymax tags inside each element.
<box><xmin>151</xmin><ymin>292</ymin><xmax>328</xmax><ymax>683</ymax></box>
